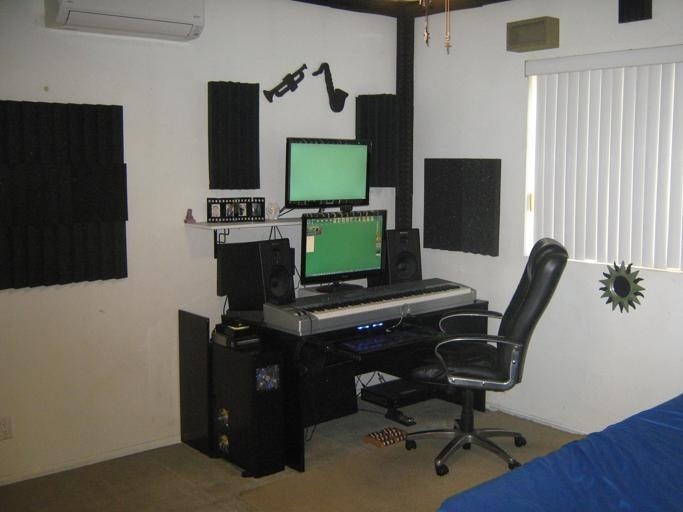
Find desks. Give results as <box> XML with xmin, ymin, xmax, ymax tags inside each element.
<box><xmin>222</xmin><ymin>278</ymin><xmax>491</xmax><ymax>473</ymax></box>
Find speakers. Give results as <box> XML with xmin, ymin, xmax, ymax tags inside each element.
<box><xmin>367</xmin><ymin>228</ymin><xmax>423</xmax><ymax>286</ymax></box>
<box><xmin>221</xmin><ymin>238</ymin><xmax>296</xmax><ymax>310</ymax></box>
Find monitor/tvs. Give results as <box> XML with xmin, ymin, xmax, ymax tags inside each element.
<box><xmin>286</xmin><ymin>136</ymin><xmax>372</xmax><ymax>210</ymax></box>
<box><xmin>299</xmin><ymin>209</ymin><xmax>387</xmax><ymax>293</ymax></box>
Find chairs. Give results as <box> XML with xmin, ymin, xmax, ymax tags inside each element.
<box><xmin>380</xmin><ymin>239</ymin><xmax>570</xmax><ymax>474</ymax></box>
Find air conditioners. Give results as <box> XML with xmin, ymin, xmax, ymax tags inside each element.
<box><xmin>43</xmin><ymin>0</ymin><xmax>206</xmax><ymax>40</ymax></box>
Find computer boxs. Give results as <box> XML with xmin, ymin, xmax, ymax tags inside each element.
<box><xmin>298</xmin><ymin>361</ymin><xmax>358</xmax><ymax>424</ymax></box>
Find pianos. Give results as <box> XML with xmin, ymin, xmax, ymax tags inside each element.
<box><xmin>264</xmin><ymin>277</ymin><xmax>477</xmax><ymax>336</ymax></box>
